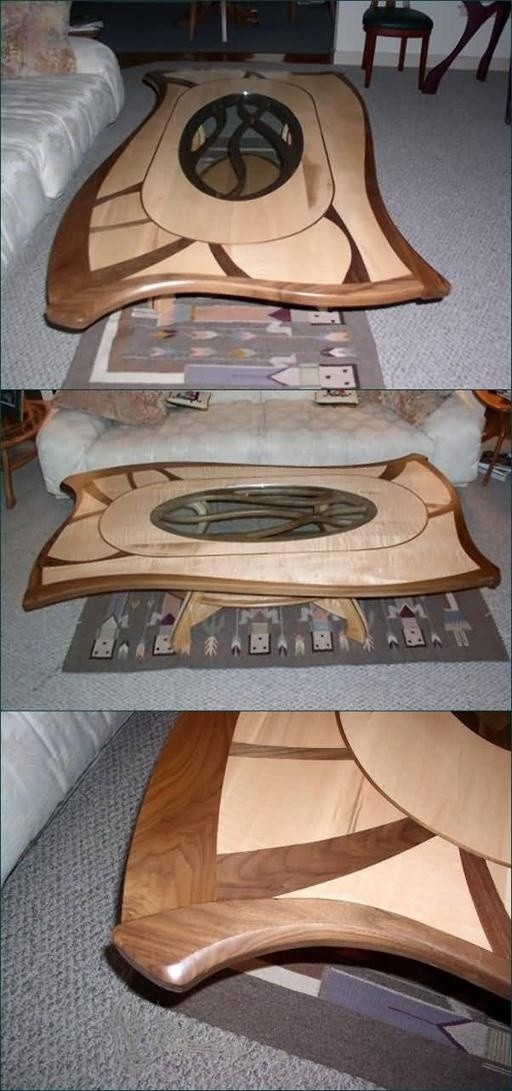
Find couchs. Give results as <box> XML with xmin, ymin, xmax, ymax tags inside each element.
<box><xmin>1</xmin><ymin>0</ymin><xmax>133</xmax><ymax>287</ymax></box>
<box><xmin>28</xmin><ymin>392</ymin><xmax>484</xmax><ymax>497</ymax></box>
<box><xmin>0</xmin><ymin>711</ymin><xmax>125</xmax><ymax>893</ymax></box>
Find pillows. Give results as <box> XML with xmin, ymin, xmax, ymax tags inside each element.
<box><xmin>0</xmin><ymin>1</ymin><xmax>79</xmax><ymax>80</ymax></box>
<box><xmin>55</xmin><ymin>388</ymin><xmax>168</xmax><ymax>423</ymax></box>
<box><xmin>354</xmin><ymin>390</ymin><xmax>464</xmax><ymax>427</ymax></box>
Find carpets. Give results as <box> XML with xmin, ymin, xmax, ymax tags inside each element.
<box><xmin>103</xmin><ymin>932</ymin><xmax>512</xmax><ymax>1089</ymax></box>
<box><xmin>48</xmin><ymin>584</ymin><xmax>511</xmax><ymax>677</ymax></box>
<box><xmin>46</xmin><ymin>274</ymin><xmax>389</xmax><ymax>386</ymax></box>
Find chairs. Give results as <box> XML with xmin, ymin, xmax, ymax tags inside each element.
<box><xmin>1</xmin><ymin>394</ymin><xmax>44</xmax><ymax>510</ymax></box>
<box><xmin>360</xmin><ymin>0</ymin><xmax>433</xmax><ymax>89</ymax></box>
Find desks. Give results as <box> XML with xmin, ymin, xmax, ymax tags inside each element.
<box><xmin>44</xmin><ymin>68</ymin><xmax>451</xmax><ymax>334</ymax></box>
<box><xmin>17</xmin><ymin>448</ymin><xmax>501</xmax><ymax>649</ymax></box>
<box><xmin>107</xmin><ymin>711</ymin><xmax>512</xmax><ymax>1003</ymax></box>
<box><xmin>473</xmin><ymin>391</ymin><xmax>511</xmax><ymax>489</ymax></box>
